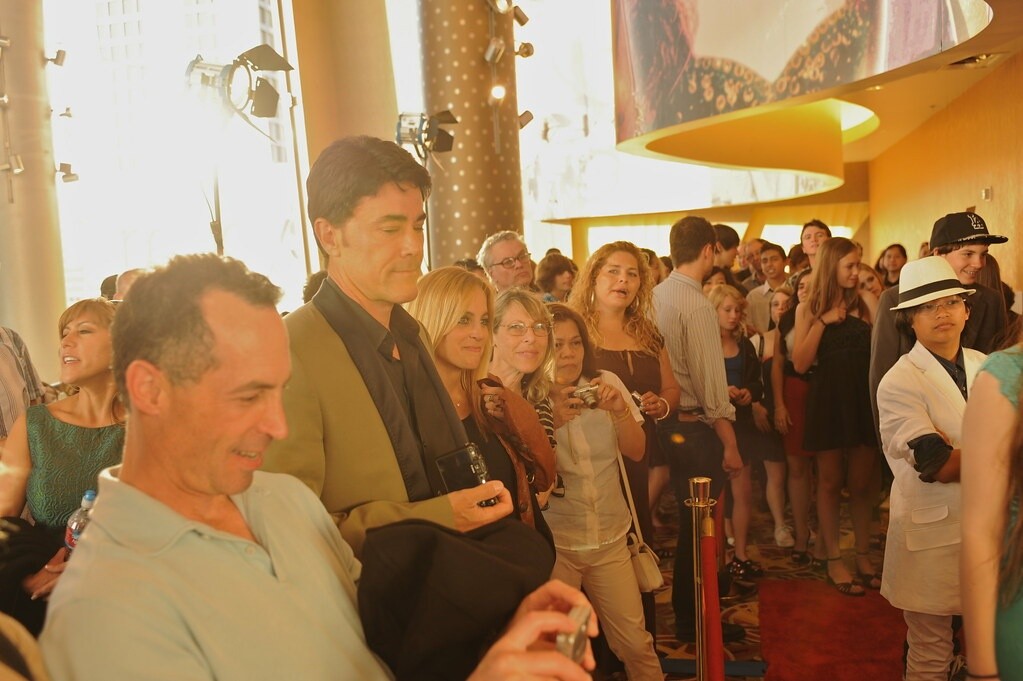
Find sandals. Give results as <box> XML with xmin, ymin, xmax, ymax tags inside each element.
<box><xmin>826</xmin><ymin>557</ymin><xmax>862</xmax><ymax>595</ymax></box>
<box><xmin>854</xmin><ymin>552</ymin><xmax>883</xmax><ymax>589</ymax></box>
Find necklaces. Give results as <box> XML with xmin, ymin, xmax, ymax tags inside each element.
<box><xmin>452</xmin><ymin>387</ymin><xmax>463</xmax><ymax>408</ymax></box>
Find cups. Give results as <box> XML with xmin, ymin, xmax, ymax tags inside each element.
<box><xmin>434</xmin><ymin>442</ymin><xmax>500</xmax><ymax>509</ymax></box>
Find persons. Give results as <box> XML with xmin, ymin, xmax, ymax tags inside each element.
<box><xmin>253</xmin><ymin>134</ymin><xmax>513</xmax><ymax>551</ymax></box>
<box><xmin>0</xmin><ymin>212</ymin><xmax>1023</xmax><ymax>681</ymax></box>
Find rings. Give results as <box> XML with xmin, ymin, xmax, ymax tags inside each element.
<box><xmin>491</xmin><ymin>411</ymin><xmax>494</xmax><ymax>414</ymax></box>
<box><xmin>488</xmin><ymin>395</ymin><xmax>495</xmax><ymax>402</ymax></box>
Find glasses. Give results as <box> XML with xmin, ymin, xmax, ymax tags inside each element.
<box><xmin>489</xmin><ymin>251</ymin><xmax>532</xmax><ymax>270</ymax></box>
<box><xmin>914</xmin><ymin>296</ymin><xmax>968</xmax><ymax>315</ymax></box>
<box><xmin>495</xmin><ymin>320</ymin><xmax>554</xmax><ymax>338</ymax></box>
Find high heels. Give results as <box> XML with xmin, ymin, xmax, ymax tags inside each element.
<box><xmin>791</xmin><ymin>529</ymin><xmax>810</xmax><ymax>562</ymax></box>
<box><xmin>811</xmin><ymin>534</ymin><xmax>827</xmax><ymax>569</ymax></box>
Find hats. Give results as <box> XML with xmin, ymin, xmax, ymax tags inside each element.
<box><xmin>889</xmin><ymin>254</ymin><xmax>976</xmax><ymax>312</ymax></box>
<box><xmin>930</xmin><ymin>211</ymin><xmax>1009</xmax><ymax>249</ymax></box>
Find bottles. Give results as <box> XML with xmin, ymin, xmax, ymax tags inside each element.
<box><xmin>63</xmin><ymin>489</ymin><xmax>96</xmax><ymax>562</ymax></box>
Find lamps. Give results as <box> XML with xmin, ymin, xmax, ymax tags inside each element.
<box><xmin>484</xmin><ymin>0</ymin><xmax>534</xmax><ymax>129</ymax></box>
<box><xmin>0</xmin><ymin>39</ymin><xmax>79</xmax><ymax>183</ymax></box>
<box><xmin>185</xmin><ymin>45</ymin><xmax>295</xmax><ymax>118</ymax></box>
<box><xmin>398</xmin><ymin>110</ymin><xmax>457</xmax><ymax>164</ymax></box>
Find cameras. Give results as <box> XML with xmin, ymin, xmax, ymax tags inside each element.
<box><xmin>555</xmin><ymin>604</ymin><xmax>591</xmax><ymax>668</ymax></box>
<box><xmin>572</xmin><ymin>382</ymin><xmax>600</xmax><ymax>410</ymax></box>
<box><xmin>630</xmin><ymin>391</ymin><xmax>650</xmax><ymax>412</ymax></box>
<box><xmin>435</xmin><ymin>443</ymin><xmax>500</xmax><ymax>506</ymax></box>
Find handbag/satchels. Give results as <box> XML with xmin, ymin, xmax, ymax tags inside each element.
<box><xmin>625</xmin><ymin>532</ymin><xmax>665</xmax><ymax>594</ymax></box>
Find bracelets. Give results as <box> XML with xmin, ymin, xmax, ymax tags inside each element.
<box><xmin>657</xmin><ymin>397</ymin><xmax>670</xmax><ymax>421</ymax></box>
<box><xmin>616</xmin><ymin>409</ymin><xmax>631</xmax><ymax>422</ymax></box>
<box><xmin>614</xmin><ymin>404</ymin><xmax>629</xmax><ymax>415</ymax></box>
<box><xmin>818</xmin><ymin>317</ymin><xmax>826</xmax><ymax>327</ymax></box>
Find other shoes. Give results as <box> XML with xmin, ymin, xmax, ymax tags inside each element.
<box><xmin>733</xmin><ymin>554</ymin><xmax>765</xmax><ymax>577</ymax></box>
<box><xmin>774</xmin><ymin>525</ymin><xmax>794</xmax><ymax>547</ymax></box>
<box><xmin>676</xmin><ymin>620</ymin><xmax>745</xmax><ymax>642</ymax></box>
<box><xmin>725</xmin><ymin>561</ymin><xmax>750</xmax><ymax>580</ymax></box>
